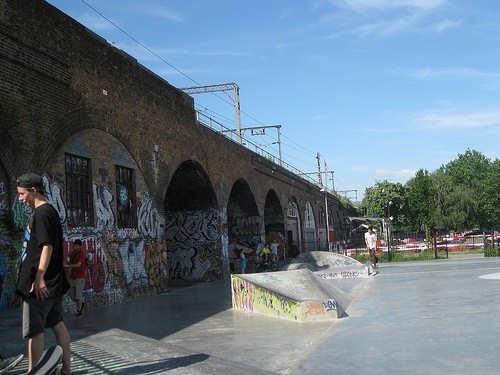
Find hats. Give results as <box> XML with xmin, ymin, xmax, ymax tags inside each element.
<box><xmin>16</xmin><ymin>172</ymin><xmax>51</xmax><ymax>194</ymax></box>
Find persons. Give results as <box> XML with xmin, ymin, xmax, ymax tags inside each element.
<box><xmin>16</xmin><ymin>173</ymin><xmax>72</xmax><ymax>375</ymax></box>
<box><xmin>63</xmin><ymin>239</ymin><xmax>87</xmax><ymax>316</ymax></box>
<box><xmin>226</xmin><ymin>240</ymin><xmax>300</xmax><ymax>274</ymax></box>
<box><xmin>364</xmin><ymin>227</ymin><xmax>379</xmax><ymax>269</ymax></box>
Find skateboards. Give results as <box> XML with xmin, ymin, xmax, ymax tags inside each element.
<box><xmin>24</xmin><ymin>345</ymin><xmax>64</xmax><ymax>375</ymax></box>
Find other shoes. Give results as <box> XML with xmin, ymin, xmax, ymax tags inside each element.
<box><xmin>74</xmin><ymin>302</ymin><xmax>85</xmax><ymax>316</ymax></box>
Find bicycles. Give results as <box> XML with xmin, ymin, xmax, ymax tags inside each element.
<box><xmin>255</xmin><ymin>253</ymin><xmax>279</xmax><ymax>273</ymax></box>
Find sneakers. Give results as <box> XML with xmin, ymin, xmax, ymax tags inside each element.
<box><xmin>0</xmin><ymin>351</ymin><xmax>24</xmax><ymax>375</ymax></box>
